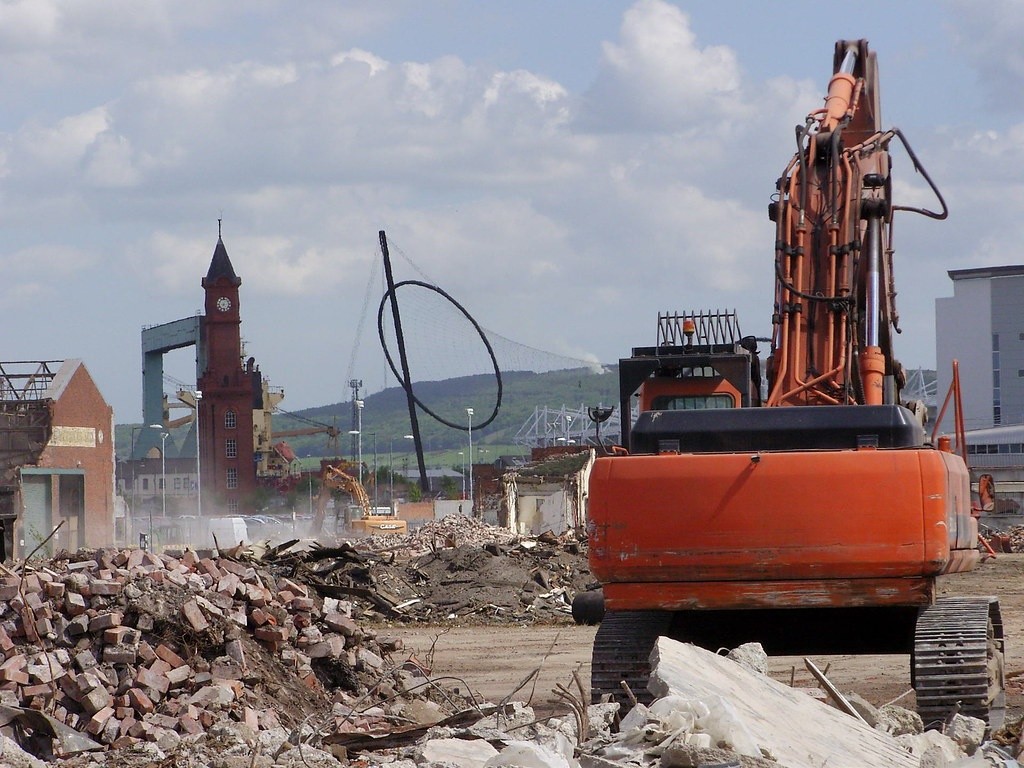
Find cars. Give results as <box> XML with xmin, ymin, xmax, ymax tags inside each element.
<box><xmin>179</xmin><ymin>513</ymin><xmax>343</xmax><ymax>543</ymax></box>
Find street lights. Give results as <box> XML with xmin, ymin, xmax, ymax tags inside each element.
<box><xmin>160</xmin><ymin>432</ymin><xmax>170</xmax><ymax>516</ymax></box>
<box><xmin>130</xmin><ymin>423</ymin><xmax>163</xmax><ymax>545</ymax></box>
<box><xmin>193</xmin><ymin>390</ymin><xmax>203</xmax><ymax>519</ymax></box>
<box><xmin>348</xmin><ymin>399</ymin><xmax>365</xmax><ymax>484</ymax></box>
<box><xmin>555</xmin><ymin>414</ymin><xmax>576</xmax><ymax>449</ymax></box>
<box><xmin>465</xmin><ymin>407</ymin><xmax>474</xmax><ymax>500</ymax></box>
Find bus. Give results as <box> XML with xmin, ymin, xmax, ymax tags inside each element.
<box><xmin>349</xmin><ymin>504</ymin><xmax>391</xmax><ymax>518</ymax></box>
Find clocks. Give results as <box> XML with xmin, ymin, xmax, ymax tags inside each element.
<box><xmin>216</xmin><ymin>296</ymin><xmax>231</xmax><ymax>312</ymax></box>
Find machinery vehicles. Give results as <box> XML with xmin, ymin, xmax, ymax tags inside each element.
<box><xmin>571</xmin><ymin>39</ymin><xmax>1008</xmax><ymax>745</ymax></box>
<box><xmin>310</xmin><ymin>464</ymin><xmax>409</xmax><ymax>538</ymax></box>
